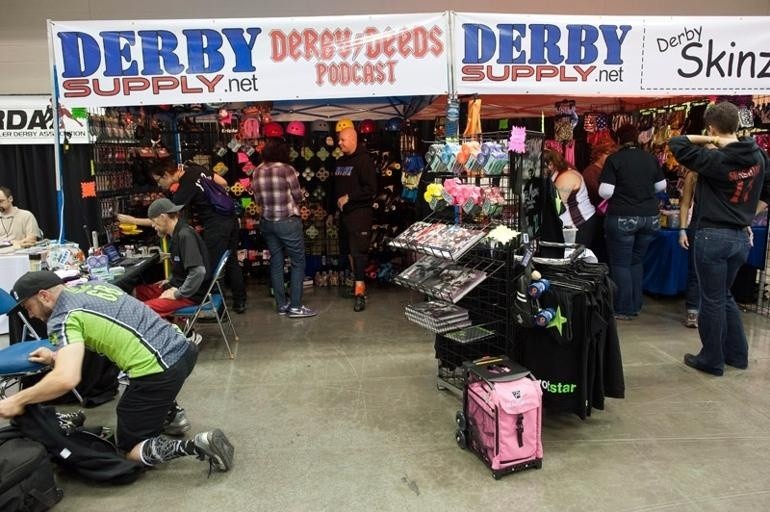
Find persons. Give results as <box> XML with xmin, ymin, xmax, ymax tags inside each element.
<box><xmin>115</xmin><ymin>161</ymin><xmax>245</xmax><ymax>324</ymax></box>
<box><xmin>325</xmin><ymin>126</ymin><xmax>377</xmax><ymax>312</ymax></box>
<box><xmin>0</xmin><ymin>185</ymin><xmax>44</xmax><ymax>248</ymax></box>
<box><xmin>131</xmin><ymin>199</ymin><xmax>210</xmax><ymax>348</ymax></box>
<box><xmin>536</xmin><ymin>104</ymin><xmax>716</xmax><ymax>327</ymax></box>
<box><xmin>0</xmin><ymin>270</ymin><xmax>234</xmax><ymax>474</ymax></box>
<box><xmin>666</xmin><ymin>103</ymin><xmax>765</xmax><ymax>376</ymax></box>
<box><xmin>252</xmin><ymin>136</ymin><xmax>319</xmax><ymax>319</ymax></box>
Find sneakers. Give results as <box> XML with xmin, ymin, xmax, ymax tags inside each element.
<box><xmin>344</xmin><ymin>289</ymin><xmax>368</xmax><ymax>312</ymax></box>
<box><xmin>684</xmin><ymin>353</ymin><xmax>723</xmax><ymax>375</ymax></box>
<box><xmin>278</xmin><ymin>301</ymin><xmax>290</xmax><ymax>316</ymax></box>
<box><xmin>232</xmin><ymin>304</ymin><xmax>245</xmax><ymax>314</ymax></box>
<box><xmin>189</xmin><ymin>332</ymin><xmax>204</xmax><ymax>345</ymax></box>
<box><xmin>163</xmin><ymin>403</ymin><xmax>191</xmax><ymax>434</ymax></box>
<box><xmin>195</xmin><ymin>428</ymin><xmax>233</xmax><ymax>472</ymax></box>
<box><xmin>686</xmin><ymin>308</ymin><xmax>698</xmax><ymax>328</ymax></box>
<box><xmin>289</xmin><ymin>306</ymin><xmax>317</xmax><ymax>318</ymax></box>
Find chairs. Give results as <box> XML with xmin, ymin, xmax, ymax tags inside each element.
<box><xmin>0</xmin><ymin>288</ymin><xmax>84</xmax><ymax>404</ymax></box>
<box><xmin>175</xmin><ymin>251</ymin><xmax>240</xmax><ymax>358</ymax></box>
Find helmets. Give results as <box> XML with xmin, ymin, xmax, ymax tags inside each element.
<box><xmin>385</xmin><ymin>118</ymin><xmax>402</xmax><ymax>132</ymax></box>
<box><xmin>264</xmin><ymin>118</ymin><xmax>375</xmax><ymax>138</ymax></box>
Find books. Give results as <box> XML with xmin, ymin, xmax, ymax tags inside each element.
<box><xmin>389</xmin><ymin>221</ymin><xmax>495</xmax><ymax>342</ymax></box>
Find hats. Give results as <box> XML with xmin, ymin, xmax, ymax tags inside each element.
<box><xmin>147</xmin><ymin>198</ymin><xmax>184</xmax><ymax>219</ymax></box>
<box><xmin>6</xmin><ymin>271</ymin><xmax>61</xmax><ymax>317</ymax></box>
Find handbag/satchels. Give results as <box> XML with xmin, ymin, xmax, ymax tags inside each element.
<box><xmin>200</xmin><ymin>175</ymin><xmax>234</xmax><ymax>215</ymax></box>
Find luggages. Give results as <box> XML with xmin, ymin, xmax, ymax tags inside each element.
<box><xmin>0</xmin><ymin>436</ymin><xmax>64</xmax><ymax>511</ymax></box>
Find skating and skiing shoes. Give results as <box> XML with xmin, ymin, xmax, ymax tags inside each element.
<box><xmin>510</xmin><ymin>275</ymin><xmax>555</xmax><ymax>328</ymax></box>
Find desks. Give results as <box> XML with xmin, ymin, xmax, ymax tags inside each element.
<box><xmin>642</xmin><ymin>230</ymin><xmax>689</xmax><ymax>303</ymax></box>
<box><xmin>743</xmin><ymin>226</ymin><xmax>767</xmax><ymax>312</ymax></box>
<box><xmin>8</xmin><ymin>257</ymin><xmax>160</xmax><ymax>408</ymax></box>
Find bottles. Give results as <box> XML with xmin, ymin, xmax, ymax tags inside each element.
<box><xmin>29</xmin><ymin>252</ymin><xmax>42</xmax><ymax>272</ymax></box>
<box><xmin>89</xmin><ymin>111</ymin><xmax>134</xmax><ymax>241</ymax></box>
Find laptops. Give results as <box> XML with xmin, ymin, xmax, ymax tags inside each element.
<box><xmin>102</xmin><ymin>242</ymin><xmax>143</xmax><ymax>267</ymax></box>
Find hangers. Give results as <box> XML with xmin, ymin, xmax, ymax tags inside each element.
<box><xmin>548</xmin><ymin>244</ymin><xmax>610</xmax><ymax>292</ymax></box>
